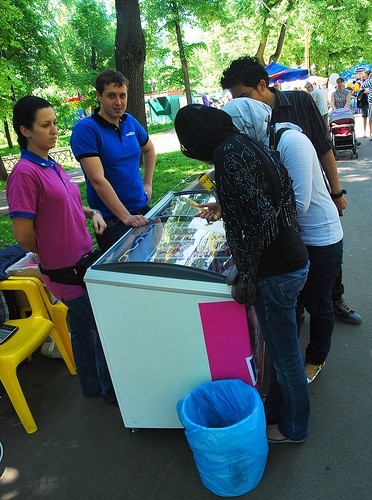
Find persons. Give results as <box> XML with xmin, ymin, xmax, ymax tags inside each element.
<box><xmin>331</xmin><ymin>78</ymin><xmax>351</xmax><ymax>154</ymax></box>
<box><xmin>346</xmin><ymin>71</ymin><xmax>372</xmax><ymax>141</ymax></box>
<box><xmin>174</xmin><ymin>105</ymin><xmax>309</xmax><ymax>443</ymax></box>
<box><xmin>222</xmin><ymin>98</ymin><xmax>343</xmax><ymax>383</ymax></box>
<box><xmin>70</xmin><ymin>70</ymin><xmax>156</xmax><ymax>251</ymax></box>
<box><xmin>6</xmin><ymin>96</ymin><xmax>123</xmax><ymax>406</ymax></box>
<box><xmin>222</xmin><ymin>58</ymin><xmax>362</xmax><ymax>325</ymax></box>
<box><xmin>305</xmin><ymin>82</ymin><xmax>331</xmax><ymax>137</ymax></box>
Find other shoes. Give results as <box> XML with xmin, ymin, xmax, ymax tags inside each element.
<box><xmin>304</xmin><ymin>360</ymin><xmax>326</xmax><ymax>385</ymax></box>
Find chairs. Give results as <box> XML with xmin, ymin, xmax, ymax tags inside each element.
<box><xmin>0</xmin><ymin>276</ymin><xmax>78</xmax><ymax>434</ymax></box>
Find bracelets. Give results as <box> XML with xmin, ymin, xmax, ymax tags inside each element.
<box><xmin>90</xmin><ymin>210</ymin><xmax>96</xmax><ymax>218</ymax></box>
<box><xmin>331</xmin><ymin>190</ymin><xmax>346</xmax><ymax>199</ymax></box>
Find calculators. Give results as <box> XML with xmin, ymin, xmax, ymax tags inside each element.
<box><xmin>0</xmin><ymin>324</ymin><xmax>18</xmax><ymax>345</ymax></box>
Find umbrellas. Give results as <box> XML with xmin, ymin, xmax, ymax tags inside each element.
<box><xmin>264</xmin><ymin>61</ymin><xmax>309</xmax><ymax>91</ymax></box>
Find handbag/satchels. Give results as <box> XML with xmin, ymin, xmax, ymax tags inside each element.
<box><xmin>74</xmin><ymin>248</ymin><xmax>104</xmax><ymax>290</ymax></box>
<box><xmin>226</xmin><ymin>265</ymin><xmax>257</xmax><ymax>307</ymax></box>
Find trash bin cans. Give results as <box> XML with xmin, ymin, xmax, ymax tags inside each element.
<box><xmin>182</xmin><ymin>379</ymin><xmax>268</xmax><ymax>497</ymax></box>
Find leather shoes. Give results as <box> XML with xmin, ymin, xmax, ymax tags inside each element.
<box><xmin>266</xmin><ymin>424</ymin><xmax>306</xmax><ymax>443</ymax></box>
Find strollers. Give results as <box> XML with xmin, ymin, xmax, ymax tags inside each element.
<box><xmin>328</xmin><ymin>108</ymin><xmax>359</xmax><ymax>160</ymax></box>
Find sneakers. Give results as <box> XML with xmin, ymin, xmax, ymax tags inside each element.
<box><xmin>333</xmin><ymin>296</ymin><xmax>363</xmax><ymax>325</ymax></box>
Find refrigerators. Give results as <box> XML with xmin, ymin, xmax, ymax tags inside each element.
<box><xmin>83</xmin><ymin>167</ymin><xmax>264</xmax><ymax>428</ymax></box>
